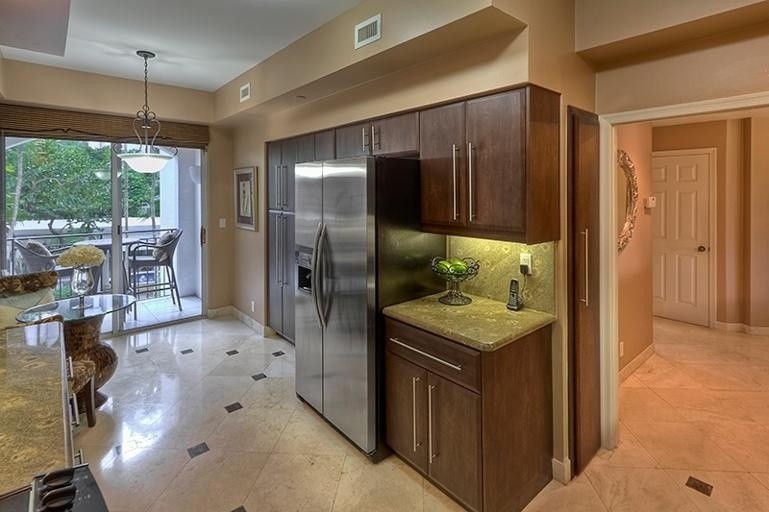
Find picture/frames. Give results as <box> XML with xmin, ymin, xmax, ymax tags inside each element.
<box><xmin>230</xmin><ymin>163</ymin><xmax>260</xmax><ymax>234</ymax></box>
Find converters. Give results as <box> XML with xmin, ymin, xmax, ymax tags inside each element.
<box><xmin>520</xmin><ymin>265</ymin><xmax>528</xmax><ymax>274</ymax></box>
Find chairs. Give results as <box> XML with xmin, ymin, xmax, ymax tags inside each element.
<box><xmin>125</xmin><ymin>227</ymin><xmax>189</xmax><ymax>322</ymax></box>
<box><xmin>12</xmin><ymin>238</ymin><xmax>75</xmax><ymax>300</ymax></box>
<box><xmin>0</xmin><ymin>270</ymin><xmax>64</xmax><ymax>331</ymax></box>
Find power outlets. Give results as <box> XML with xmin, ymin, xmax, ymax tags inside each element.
<box><xmin>519</xmin><ymin>253</ymin><xmax>532</xmax><ymax>275</ymax></box>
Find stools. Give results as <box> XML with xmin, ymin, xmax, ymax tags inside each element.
<box><xmin>66</xmin><ymin>356</ymin><xmax>97</xmax><ymax>430</ymax></box>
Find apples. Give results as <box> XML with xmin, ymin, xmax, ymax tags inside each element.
<box><xmin>437</xmin><ymin>261</ymin><xmax>453</xmax><ymax>273</ymax></box>
<box><xmin>449</xmin><ymin>258</ymin><xmax>469</xmax><ymax>273</ymax></box>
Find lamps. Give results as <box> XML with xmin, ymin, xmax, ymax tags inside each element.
<box><xmin>112</xmin><ymin>50</ymin><xmax>179</xmax><ymax>175</ymax></box>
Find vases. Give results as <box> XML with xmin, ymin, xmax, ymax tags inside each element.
<box><xmin>69</xmin><ymin>266</ymin><xmax>96</xmax><ymax>313</ymax></box>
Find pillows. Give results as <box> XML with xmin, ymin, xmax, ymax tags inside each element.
<box><xmin>25</xmin><ymin>240</ymin><xmax>57</xmax><ymax>272</ymax></box>
<box><xmin>150</xmin><ymin>231</ymin><xmax>177</xmax><ymax>262</ymax></box>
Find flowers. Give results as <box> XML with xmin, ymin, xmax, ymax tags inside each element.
<box><xmin>58</xmin><ymin>245</ymin><xmax>106</xmax><ymax>272</ymax></box>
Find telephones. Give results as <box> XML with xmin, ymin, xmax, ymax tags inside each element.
<box><xmin>506</xmin><ymin>279</ymin><xmax>523</xmax><ymax>310</ymax></box>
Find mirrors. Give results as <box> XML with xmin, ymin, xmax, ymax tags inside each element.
<box><xmin>616</xmin><ymin>147</ymin><xmax>642</xmax><ymax>257</ymax></box>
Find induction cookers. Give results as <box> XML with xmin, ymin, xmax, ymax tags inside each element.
<box><xmin>0</xmin><ymin>461</ymin><xmax>110</xmax><ymax>512</ymax></box>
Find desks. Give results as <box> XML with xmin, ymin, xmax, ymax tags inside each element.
<box><xmin>72</xmin><ymin>236</ymin><xmax>141</xmax><ymax>316</ymax></box>
<box><xmin>16</xmin><ymin>291</ymin><xmax>136</xmax><ymax>416</ymax></box>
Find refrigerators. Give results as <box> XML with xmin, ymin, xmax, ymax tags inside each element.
<box><xmin>294</xmin><ymin>157</ymin><xmax>449</xmax><ymax>455</ymax></box>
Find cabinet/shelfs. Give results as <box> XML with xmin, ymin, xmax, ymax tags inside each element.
<box><xmin>418</xmin><ymin>82</ymin><xmax>562</xmax><ymax>246</ymax></box>
<box><xmin>380</xmin><ymin>315</ymin><xmax>555</xmax><ymax>512</ymax></box>
<box><xmin>267</xmin><ymin>135</ymin><xmax>298</xmax><ymax>213</ymax></box>
<box><xmin>267</xmin><ymin>210</ymin><xmax>296</xmax><ymax>346</ymax></box>
<box><xmin>336</xmin><ymin>109</ymin><xmax>418</xmax><ymax>161</ymax></box>
<box><xmin>298</xmin><ymin>127</ymin><xmax>336</xmax><ymax>163</ymax></box>
<box><xmin>1</xmin><ymin>322</ymin><xmax>84</xmax><ymax>493</ymax></box>
